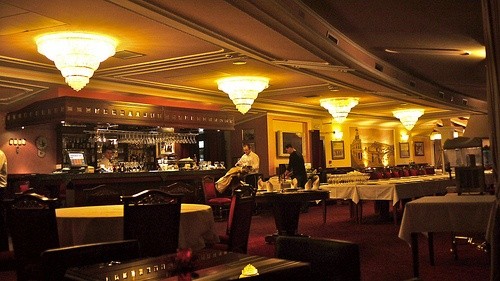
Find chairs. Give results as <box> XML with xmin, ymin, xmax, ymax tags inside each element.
<box><xmin>451</xmin><ymin>166</ymin><xmax>484</xmax><ymax>261</ymax></box>
<box><xmin>370</xmin><ymin>163</ymin><xmax>433</xmax><ymax>216</ymax></box>
<box><xmin>3</xmin><ymin>175</ymin><xmax>364</xmax><ymax>281</ymax></box>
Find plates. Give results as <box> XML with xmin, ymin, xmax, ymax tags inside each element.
<box><xmin>258</xmin><ymin>188</ymin><xmax>323</xmax><ymax>193</ymax></box>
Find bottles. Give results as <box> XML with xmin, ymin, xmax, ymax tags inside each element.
<box><xmin>117</xmin><ymin>144</ymin><xmax>155</xmax><ymax>173</ymax></box>
<box><xmin>64</xmin><ymin>136</ymin><xmax>94</xmax><ymax>148</ymax></box>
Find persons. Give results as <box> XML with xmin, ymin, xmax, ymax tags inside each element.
<box><xmin>145</xmin><ymin>143</ymin><xmax>161</xmax><ymax>169</ymax></box>
<box><xmin>235</xmin><ymin>143</ymin><xmax>260</xmax><ymax>173</ymax></box>
<box><xmin>0</xmin><ymin>149</ymin><xmax>8</xmax><ymax>250</ymax></box>
<box><xmin>281</xmin><ymin>144</ymin><xmax>308</xmax><ymax>213</ymax></box>
<box><xmin>98</xmin><ymin>146</ymin><xmax>112</xmax><ymax>168</ymax></box>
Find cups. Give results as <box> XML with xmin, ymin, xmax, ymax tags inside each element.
<box><xmin>20</xmin><ymin>185</ymin><xmax>28</xmax><ymax>192</ymax></box>
<box><xmin>176</xmin><ymin>247</ymin><xmax>191</xmax><ymax>281</ymax></box>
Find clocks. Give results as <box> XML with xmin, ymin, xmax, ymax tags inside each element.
<box><xmin>35</xmin><ymin>136</ymin><xmax>49</xmax><ymax>157</ymax></box>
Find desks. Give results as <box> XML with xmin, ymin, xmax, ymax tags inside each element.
<box><xmin>257</xmin><ymin>187</ymin><xmax>329</xmax><ymax>239</ymax></box>
<box><xmin>55</xmin><ymin>203</ymin><xmax>221</xmax><ymax>249</ymax></box>
<box><xmin>398</xmin><ymin>194</ymin><xmax>493</xmax><ymax>278</ymax></box>
<box><xmin>66</xmin><ymin>248</ymin><xmax>313</xmax><ymax>281</ymax></box>
<box><xmin>320</xmin><ymin>172</ymin><xmax>456</xmax><ymax>224</ymax></box>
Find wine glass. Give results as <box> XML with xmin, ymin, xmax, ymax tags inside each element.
<box><xmin>328</xmin><ymin>173</ymin><xmax>371</xmax><ymax>186</ymax></box>
<box><xmin>88</xmin><ymin>130</ymin><xmax>197</xmax><ymax>144</ymax></box>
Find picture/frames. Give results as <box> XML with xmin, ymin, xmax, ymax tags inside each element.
<box><xmin>399</xmin><ymin>143</ymin><xmax>410</xmax><ymax>158</ymax></box>
<box><xmin>415</xmin><ymin>142</ymin><xmax>424</xmax><ymax>156</ymax></box>
<box><xmin>241</xmin><ymin>128</ymin><xmax>256</xmax><ymax>154</ymax></box>
<box><xmin>331</xmin><ymin>141</ymin><xmax>344</xmax><ymax>159</ymax></box>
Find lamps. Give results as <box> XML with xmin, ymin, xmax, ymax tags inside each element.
<box><xmin>218</xmin><ymin>78</ymin><xmax>269</xmax><ymax>114</ymax></box>
<box><xmin>36</xmin><ymin>32</ymin><xmax>116</xmax><ymax>91</ymax></box>
<box><xmin>321</xmin><ymin>98</ymin><xmax>359</xmax><ymax>123</ymax></box>
<box><xmin>393</xmin><ymin>110</ymin><xmax>424</xmax><ymax>131</ymax></box>
